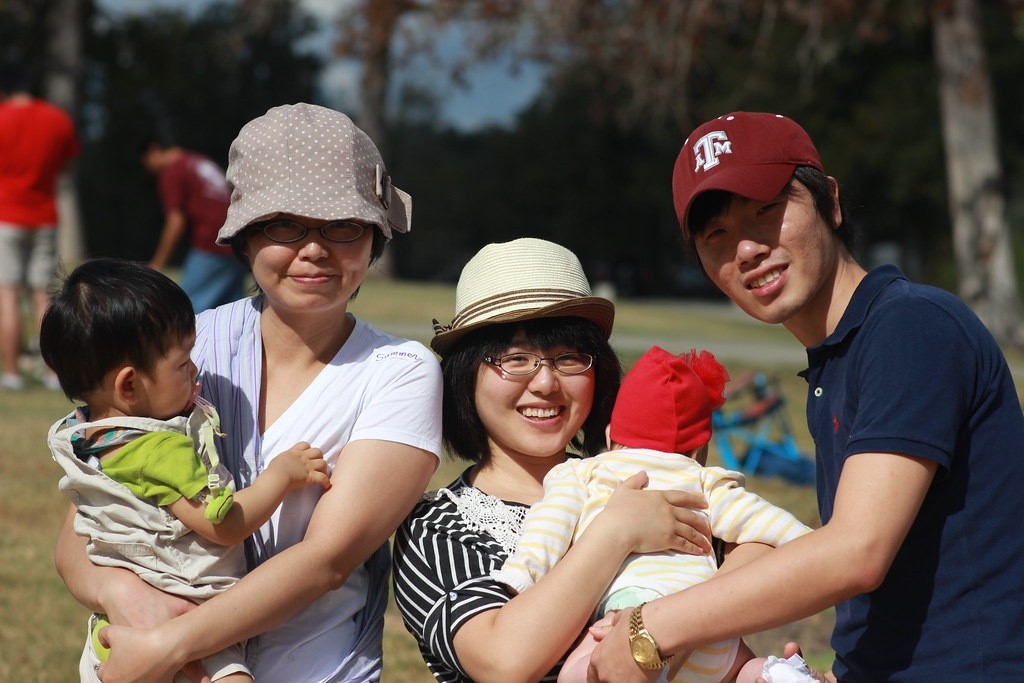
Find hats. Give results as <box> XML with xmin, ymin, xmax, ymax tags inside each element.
<box><xmin>608</xmin><ymin>345</ymin><xmax>732</xmax><ymax>453</ymax></box>
<box><xmin>214</xmin><ymin>103</ymin><xmax>413</xmax><ymax>247</ymax></box>
<box><xmin>428</xmin><ymin>238</ymin><xmax>615</xmax><ymax>356</ymax></box>
<box><xmin>671</xmin><ymin>110</ymin><xmax>825</xmax><ymax>241</ymax></box>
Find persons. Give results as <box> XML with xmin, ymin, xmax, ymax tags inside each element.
<box><xmin>36</xmin><ymin>261</ymin><xmax>333</xmax><ymax>683</ymax></box>
<box><xmin>0</xmin><ymin>64</ymin><xmax>81</xmax><ymax>394</ymax></box>
<box><xmin>52</xmin><ymin>99</ymin><xmax>445</xmax><ymax>682</ymax></box>
<box><xmin>134</xmin><ymin>124</ymin><xmax>240</xmax><ymax>315</ymax></box>
<box><xmin>579</xmin><ymin>111</ymin><xmax>1024</xmax><ymax>683</ymax></box>
<box><xmin>394</xmin><ymin>238</ymin><xmax>835</xmax><ymax>683</ymax></box>
<box><xmin>492</xmin><ymin>345</ymin><xmax>832</xmax><ymax>683</ymax></box>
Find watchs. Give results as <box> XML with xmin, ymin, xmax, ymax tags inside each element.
<box><xmin>625</xmin><ymin>601</ymin><xmax>676</xmax><ymax>670</ymax></box>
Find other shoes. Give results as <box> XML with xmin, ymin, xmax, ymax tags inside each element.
<box><xmin>2</xmin><ymin>372</ymin><xmax>25</xmax><ymax>390</ymax></box>
<box><xmin>33</xmin><ymin>370</ymin><xmax>63</xmax><ymax>391</ymax></box>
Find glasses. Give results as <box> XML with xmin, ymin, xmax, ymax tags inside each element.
<box><xmin>480</xmin><ymin>352</ymin><xmax>602</xmax><ymax>375</ymax></box>
<box><xmin>245</xmin><ymin>220</ymin><xmax>373</xmax><ymax>243</ymax></box>
